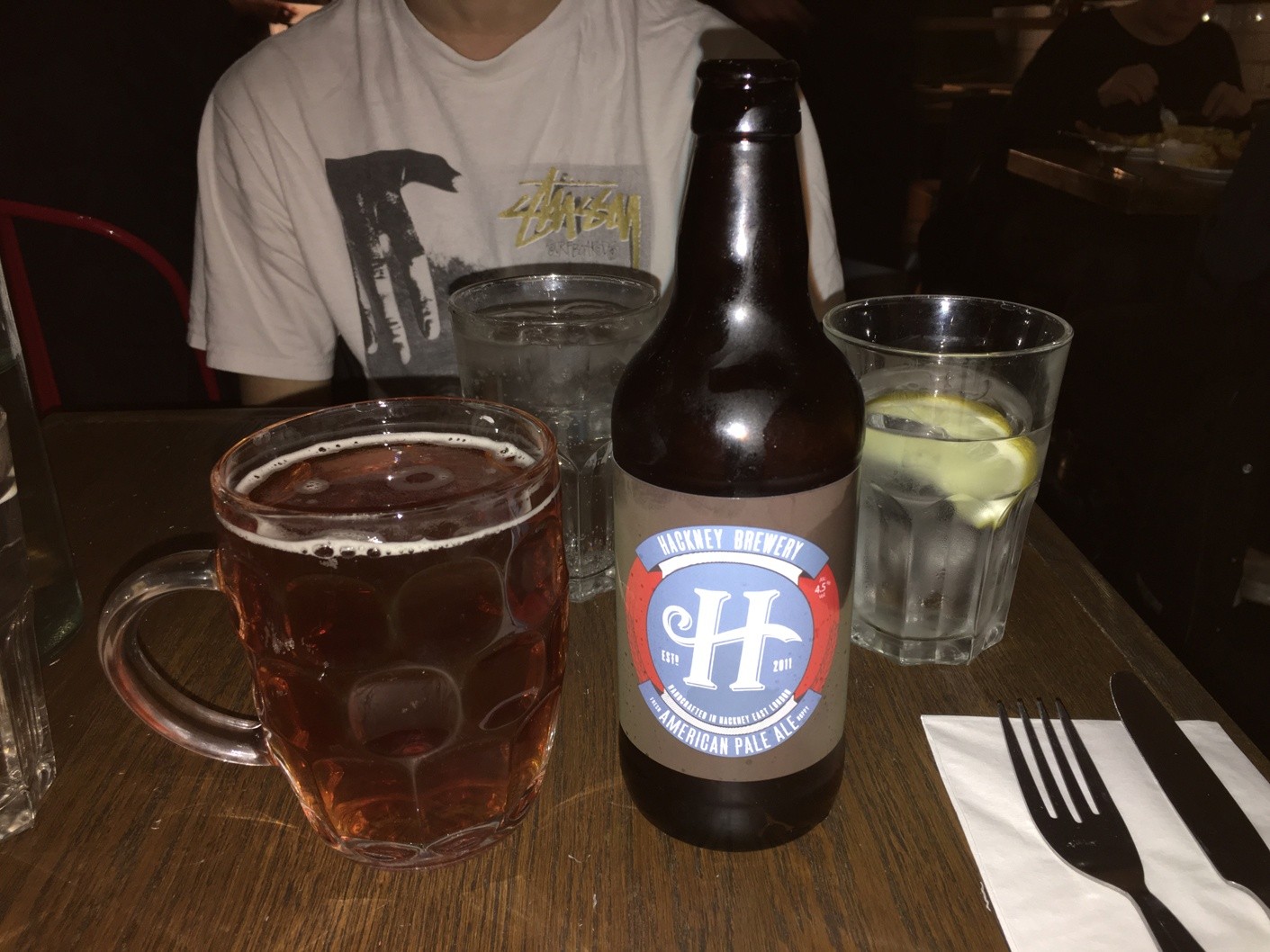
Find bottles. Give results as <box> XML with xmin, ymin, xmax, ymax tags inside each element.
<box><xmin>0</xmin><ymin>295</ymin><xmax>84</xmax><ymax>666</ymax></box>
<box><xmin>610</xmin><ymin>57</ymin><xmax>864</xmax><ymax>851</ymax></box>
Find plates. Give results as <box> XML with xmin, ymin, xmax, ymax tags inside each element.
<box><xmin>1086</xmin><ymin>126</ymin><xmax>1239</xmax><ymax>180</ymax></box>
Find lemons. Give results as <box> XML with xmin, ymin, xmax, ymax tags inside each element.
<box><xmin>859</xmin><ymin>389</ymin><xmax>1037</xmax><ymax>532</ymax></box>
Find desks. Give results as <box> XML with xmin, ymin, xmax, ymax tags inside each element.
<box><xmin>0</xmin><ymin>409</ymin><xmax>1270</xmax><ymax>952</ymax></box>
<box><xmin>1005</xmin><ymin>148</ymin><xmax>1227</xmax><ymax>214</ymax></box>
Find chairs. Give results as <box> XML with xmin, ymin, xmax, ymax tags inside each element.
<box><xmin>0</xmin><ymin>200</ymin><xmax>223</xmax><ymax>413</ymax></box>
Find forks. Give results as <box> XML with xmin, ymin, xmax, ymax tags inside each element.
<box><xmin>995</xmin><ymin>697</ymin><xmax>1206</xmax><ymax>952</ymax></box>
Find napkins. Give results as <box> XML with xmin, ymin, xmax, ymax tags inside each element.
<box><xmin>922</xmin><ymin>714</ymin><xmax>1270</xmax><ymax>952</ymax></box>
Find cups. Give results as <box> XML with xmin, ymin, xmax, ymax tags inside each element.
<box><xmin>97</xmin><ymin>395</ymin><xmax>569</xmax><ymax>869</ymax></box>
<box><xmin>820</xmin><ymin>293</ymin><xmax>1070</xmax><ymax>663</ymax></box>
<box><xmin>448</xmin><ymin>273</ymin><xmax>660</xmax><ymax>604</ymax></box>
<box><xmin>0</xmin><ymin>405</ymin><xmax>58</xmax><ymax>841</ymax></box>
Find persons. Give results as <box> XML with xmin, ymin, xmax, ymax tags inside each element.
<box><xmin>187</xmin><ymin>1</ymin><xmax>846</xmax><ymax>410</ymax></box>
<box><xmin>1014</xmin><ymin>1</ymin><xmax>1253</xmax><ymax>135</ymax></box>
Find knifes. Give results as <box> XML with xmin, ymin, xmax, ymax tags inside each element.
<box><xmin>1108</xmin><ymin>670</ymin><xmax>1270</xmax><ymax>910</ymax></box>
